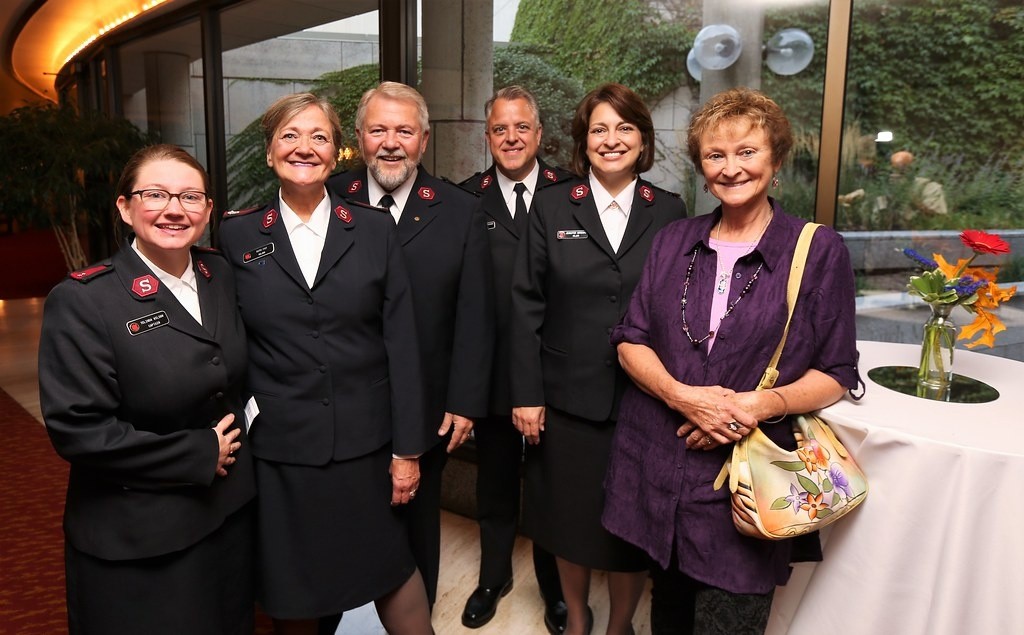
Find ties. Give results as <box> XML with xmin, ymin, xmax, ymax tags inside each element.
<box><xmin>512</xmin><ymin>183</ymin><xmax>528</xmax><ymax>234</ymax></box>
<box><xmin>381</xmin><ymin>194</ymin><xmax>393</xmax><ymax>210</ymax></box>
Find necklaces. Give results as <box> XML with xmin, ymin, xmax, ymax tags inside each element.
<box><xmin>679</xmin><ymin>246</ymin><xmax>763</xmax><ymax>346</ymax></box>
<box><xmin>717</xmin><ymin>209</ymin><xmax>772</xmax><ymax>293</ymax></box>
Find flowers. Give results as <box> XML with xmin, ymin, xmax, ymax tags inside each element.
<box><xmin>894</xmin><ymin>228</ymin><xmax>1017</xmax><ymax>382</ymax></box>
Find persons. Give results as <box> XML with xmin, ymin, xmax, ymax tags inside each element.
<box><xmin>325</xmin><ymin>81</ymin><xmax>494</xmax><ymax>618</ymax></box>
<box><xmin>837</xmin><ymin>145</ymin><xmax>948</xmax><ymax>231</ymax></box>
<box><xmin>600</xmin><ymin>89</ymin><xmax>866</xmax><ymax>635</ymax></box>
<box><xmin>509</xmin><ymin>82</ymin><xmax>688</xmax><ymax>635</ymax></box>
<box><xmin>38</xmin><ymin>143</ymin><xmax>257</xmax><ymax>635</ymax></box>
<box><xmin>6</xmin><ymin>213</ymin><xmax>28</xmax><ymax>235</ymax></box>
<box><xmin>216</xmin><ymin>93</ymin><xmax>435</xmax><ymax>635</ymax></box>
<box><xmin>457</xmin><ymin>85</ymin><xmax>584</xmax><ymax>635</ymax></box>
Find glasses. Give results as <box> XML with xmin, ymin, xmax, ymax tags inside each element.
<box><xmin>130</xmin><ymin>189</ymin><xmax>208</xmax><ymax>213</ymax></box>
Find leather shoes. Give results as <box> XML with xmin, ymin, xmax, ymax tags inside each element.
<box><xmin>539</xmin><ymin>585</ymin><xmax>568</xmax><ymax>635</ymax></box>
<box><xmin>461</xmin><ymin>569</ymin><xmax>514</xmax><ymax>628</ymax></box>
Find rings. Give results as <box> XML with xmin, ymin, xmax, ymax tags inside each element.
<box><xmin>704</xmin><ymin>434</ymin><xmax>715</xmax><ymax>444</ymax></box>
<box><xmin>230</xmin><ymin>444</ymin><xmax>233</xmax><ymax>453</ymax></box>
<box><xmin>410</xmin><ymin>490</ymin><xmax>416</xmax><ymax>496</ymax></box>
<box><xmin>466</xmin><ymin>432</ymin><xmax>469</xmax><ymax>436</ymax></box>
<box><xmin>728</xmin><ymin>420</ymin><xmax>742</xmax><ymax>433</ymax></box>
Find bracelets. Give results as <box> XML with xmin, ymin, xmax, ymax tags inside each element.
<box><xmin>763</xmin><ymin>389</ymin><xmax>788</xmax><ymax>423</ymax></box>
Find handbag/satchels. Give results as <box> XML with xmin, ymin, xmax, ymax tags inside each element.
<box><xmin>712</xmin><ymin>413</ymin><xmax>870</xmax><ymax>542</ymax></box>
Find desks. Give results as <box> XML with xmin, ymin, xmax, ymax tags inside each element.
<box><xmin>764</xmin><ymin>340</ymin><xmax>1023</xmax><ymax>635</ymax></box>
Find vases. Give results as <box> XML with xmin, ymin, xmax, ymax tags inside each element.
<box><xmin>917</xmin><ymin>304</ymin><xmax>957</xmax><ymax>388</ymax></box>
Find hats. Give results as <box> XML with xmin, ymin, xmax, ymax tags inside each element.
<box><xmin>887</xmin><ymin>151</ymin><xmax>918</xmax><ymax>177</ymax></box>
<box><xmin>855</xmin><ymin>134</ymin><xmax>878</xmax><ymax>166</ymax></box>
